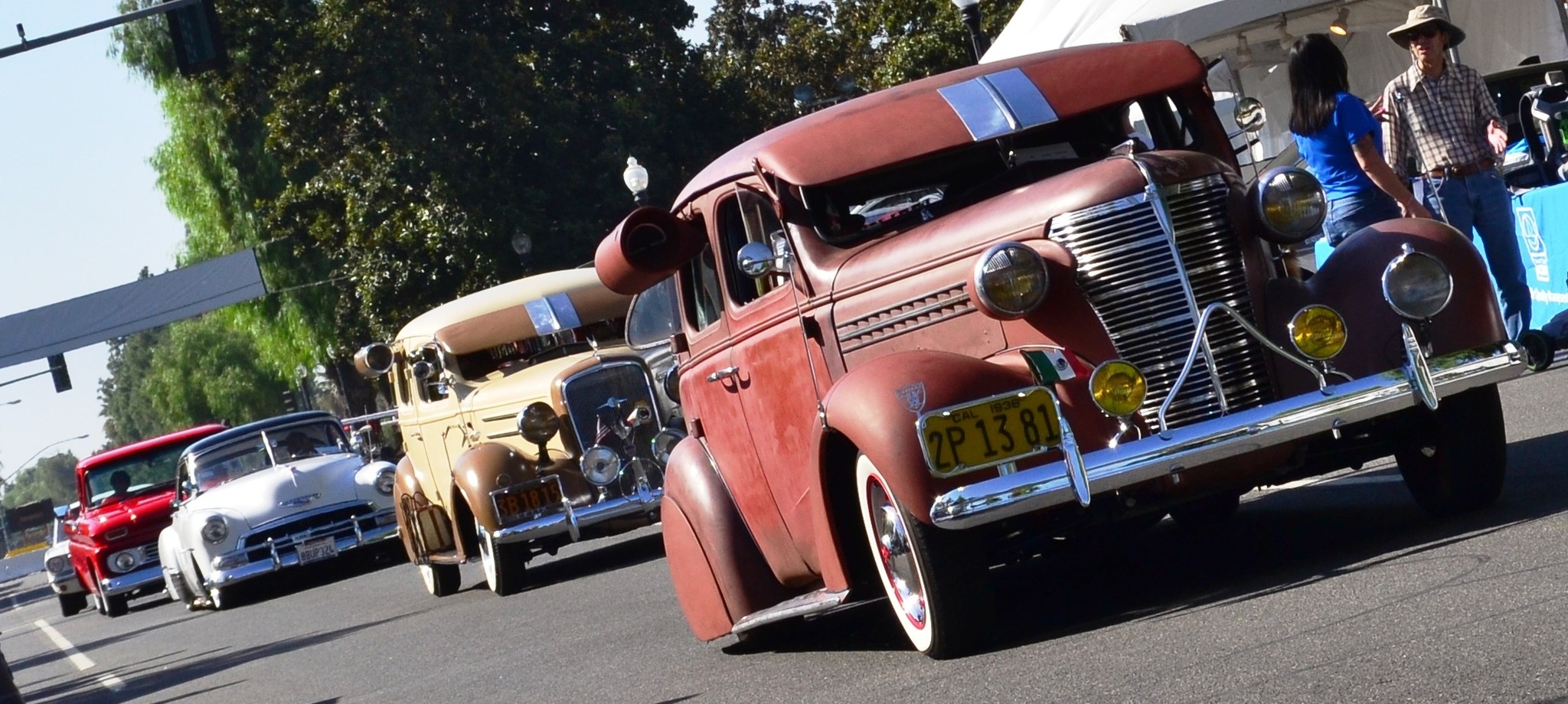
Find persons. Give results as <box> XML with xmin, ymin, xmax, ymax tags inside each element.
<box><xmin>1287</xmin><ymin>33</ymin><xmax>1435</xmax><ymax>247</ymax></box>
<box><xmin>110</xmin><ymin>470</ymin><xmax>136</xmax><ymax>498</ymax></box>
<box><xmin>1381</xmin><ymin>5</ymin><xmax>1532</xmax><ymax>343</ymax></box>
<box><xmin>286</xmin><ymin>431</ymin><xmax>314</xmax><ymax>459</ymax></box>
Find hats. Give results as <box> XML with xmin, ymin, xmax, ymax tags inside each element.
<box><xmin>1386</xmin><ymin>4</ymin><xmax>1467</xmax><ymax>52</ymax></box>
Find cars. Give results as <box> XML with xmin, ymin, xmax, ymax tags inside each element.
<box><xmin>157</xmin><ymin>410</ymin><xmax>397</xmax><ymax>613</ymax></box>
<box><xmin>1311</xmin><ymin>55</ymin><xmax>1568</xmax><ymax>374</ymax></box>
<box><xmin>594</xmin><ymin>35</ymin><xmax>1527</xmax><ymax>661</ymax></box>
<box><xmin>353</xmin><ymin>268</ymin><xmax>716</xmax><ymax>598</ymax></box>
<box><xmin>63</xmin><ymin>423</ymin><xmax>243</xmax><ymax>618</ymax></box>
<box><xmin>44</xmin><ymin>484</ymin><xmax>156</xmax><ymax>618</ymax></box>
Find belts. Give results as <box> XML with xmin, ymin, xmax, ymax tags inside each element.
<box><xmin>1422</xmin><ymin>156</ymin><xmax>1495</xmax><ymax>178</ymax></box>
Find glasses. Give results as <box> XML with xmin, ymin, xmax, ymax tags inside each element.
<box><xmin>1406</xmin><ymin>27</ymin><xmax>1443</xmax><ymax>41</ymax></box>
